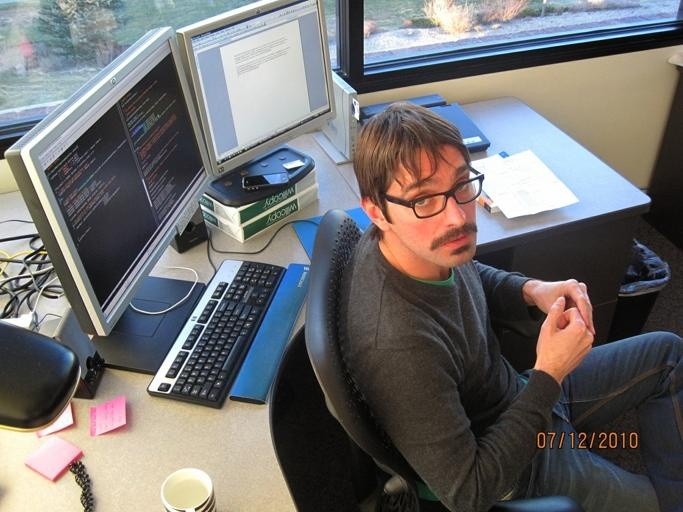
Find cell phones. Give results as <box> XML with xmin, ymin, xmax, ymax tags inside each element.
<box><xmin>242</xmin><ymin>172</ymin><xmax>289</xmax><ymax>189</ymax></box>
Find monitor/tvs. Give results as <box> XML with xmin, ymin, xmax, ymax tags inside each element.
<box><xmin>176</xmin><ymin>0</ymin><xmax>337</xmax><ymax>207</ymax></box>
<box><xmin>4</xmin><ymin>26</ymin><xmax>214</xmax><ymax>375</ymax></box>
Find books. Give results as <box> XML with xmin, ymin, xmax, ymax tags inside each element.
<box><xmin>360</xmin><ymin>95</ymin><xmax>511</xmax><ymax>213</ymax></box>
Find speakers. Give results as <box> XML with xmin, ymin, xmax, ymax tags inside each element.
<box><xmin>39</xmin><ymin>307</ymin><xmax>104</xmax><ymax>399</ymax></box>
<box><xmin>170</xmin><ymin>201</ymin><xmax>208</xmax><ymax>254</ymax></box>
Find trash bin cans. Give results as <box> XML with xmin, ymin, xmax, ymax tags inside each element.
<box><xmin>609</xmin><ymin>239</ymin><xmax>670</xmax><ymax>338</ymax></box>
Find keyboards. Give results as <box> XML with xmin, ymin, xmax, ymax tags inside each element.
<box><xmin>146</xmin><ymin>259</ymin><xmax>287</xmax><ymax>409</ymax></box>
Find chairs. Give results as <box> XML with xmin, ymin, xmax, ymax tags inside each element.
<box><xmin>299</xmin><ymin>204</ymin><xmax>595</xmax><ymax>512</ymax></box>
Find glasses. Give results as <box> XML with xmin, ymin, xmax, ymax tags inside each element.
<box><xmin>383</xmin><ymin>164</ymin><xmax>485</xmax><ymax>218</ymax></box>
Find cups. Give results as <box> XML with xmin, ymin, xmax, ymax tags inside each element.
<box><xmin>159</xmin><ymin>467</ymin><xmax>215</xmax><ymax>512</ymax></box>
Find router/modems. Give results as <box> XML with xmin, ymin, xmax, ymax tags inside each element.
<box><xmin>322</xmin><ymin>70</ymin><xmax>359</xmax><ymax>163</ymax></box>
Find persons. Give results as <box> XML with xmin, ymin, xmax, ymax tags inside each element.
<box><xmin>335</xmin><ymin>97</ymin><xmax>683</xmax><ymax>512</ymax></box>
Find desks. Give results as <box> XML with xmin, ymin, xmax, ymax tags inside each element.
<box><xmin>0</xmin><ymin>94</ymin><xmax>653</xmax><ymax>512</ymax></box>
